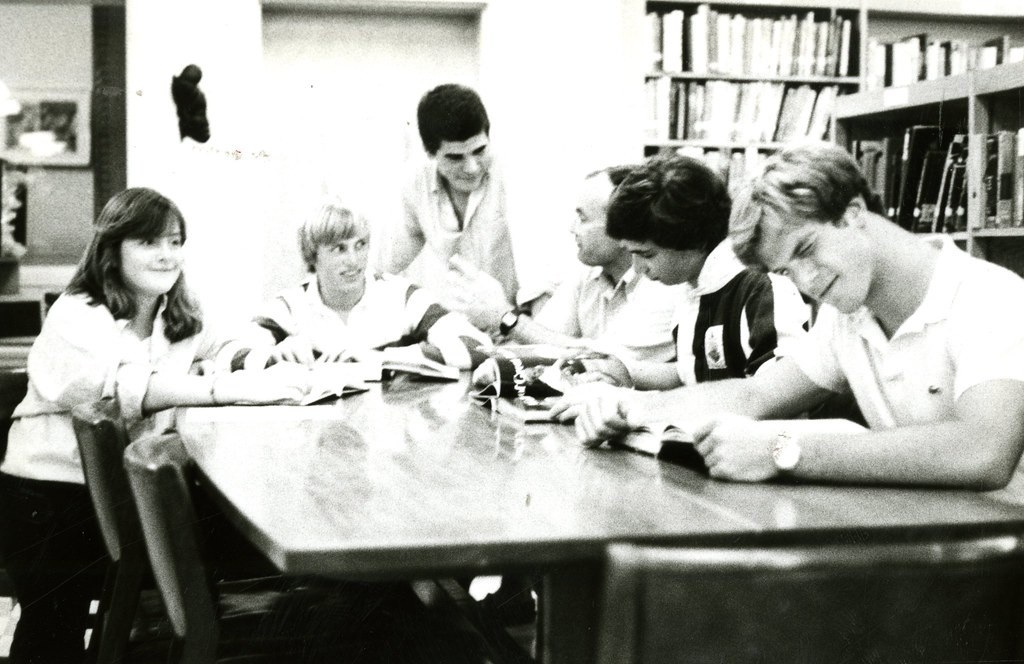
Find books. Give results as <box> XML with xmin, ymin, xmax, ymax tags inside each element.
<box><xmin>617</xmin><ymin>416</ymin><xmax>875</xmax><ymax>460</ymax></box>
<box><xmin>866</xmin><ymin>30</ymin><xmax>1024</xmax><ymax>98</ymax></box>
<box><xmin>214</xmin><ymin>341</ymin><xmax>621</xmax><ymax>427</ymax></box>
<box><xmin>642</xmin><ymin>1</ymin><xmax>858</xmax><ymax>84</ymax></box>
<box><xmin>652</xmin><ymin>73</ymin><xmax>843</xmax><ymax>161</ymax></box>
<box><xmin>846</xmin><ymin>123</ymin><xmax>1023</xmax><ymax>234</ymax></box>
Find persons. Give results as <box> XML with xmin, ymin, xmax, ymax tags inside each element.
<box><xmin>213</xmin><ymin>204</ymin><xmax>490</xmax><ymax>384</ymax></box>
<box><xmin>573</xmin><ymin>143</ymin><xmax>1018</xmax><ymax>499</ymax></box>
<box><xmin>444</xmin><ymin>161</ymin><xmax>699</xmax><ymax>363</ymax></box>
<box><xmin>538</xmin><ymin>150</ymin><xmax>811</xmax><ymax>418</ymax></box>
<box><xmin>370</xmin><ymin>85</ymin><xmax>569</xmax><ymax>327</ymax></box>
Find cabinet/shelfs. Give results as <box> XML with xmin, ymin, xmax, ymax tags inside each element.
<box><xmin>831</xmin><ymin>59</ymin><xmax>1024</xmax><ymax>258</ymax></box>
<box><xmin>640</xmin><ymin>0</ymin><xmax>1024</xmax><ymax>157</ymax></box>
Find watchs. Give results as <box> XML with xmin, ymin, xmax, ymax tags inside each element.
<box><xmin>497</xmin><ymin>309</ymin><xmax>524</xmax><ymax>337</ymax></box>
<box><xmin>772</xmin><ymin>422</ymin><xmax>801</xmax><ymax>477</ymax></box>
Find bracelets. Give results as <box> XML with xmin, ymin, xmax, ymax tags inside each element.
<box><xmin>0</xmin><ymin>187</ymin><xmax>326</xmax><ymax>664</ymax></box>
<box><xmin>207</xmin><ymin>384</ymin><xmax>219</xmax><ymax>406</ymax></box>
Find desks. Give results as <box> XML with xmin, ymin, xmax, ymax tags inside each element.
<box><xmin>175</xmin><ymin>367</ymin><xmax>1024</xmax><ymax>664</ymax></box>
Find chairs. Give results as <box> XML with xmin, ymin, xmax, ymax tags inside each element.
<box><xmin>69</xmin><ymin>406</ymin><xmax>285</xmax><ymax>664</ymax></box>
<box><xmin>122</xmin><ymin>424</ymin><xmax>382</xmax><ymax>663</ymax></box>
<box><xmin>592</xmin><ymin>536</ymin><xmax>1024</xmax><ymax>662</ymax></box>
